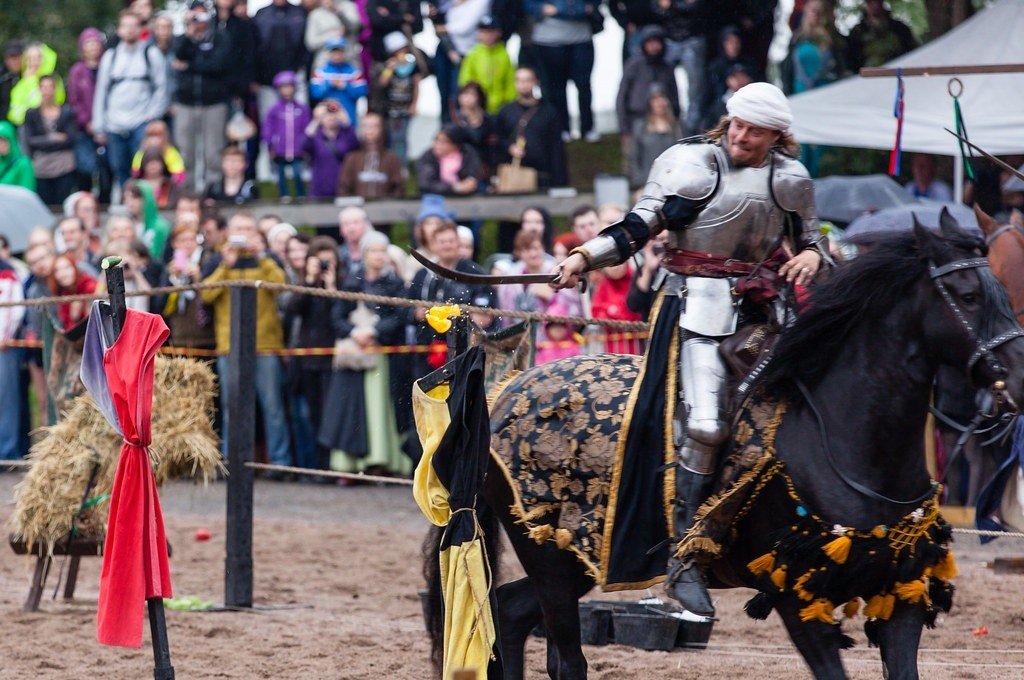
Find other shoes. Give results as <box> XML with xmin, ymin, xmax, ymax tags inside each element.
<box><xmin>584</xmin><ymin>130</ymin><xmax>600</xmax><ymax>142</ymax></box>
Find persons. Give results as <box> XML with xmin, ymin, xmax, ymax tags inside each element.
<box><xmin>0</xmin><ymin>0</ymin><xmax>1023</xmax><ymax>266</ymax></box>
<box><xmin>201</xmin><ymin>209</ymin><xmax>287</xmax><ymax>482</ymax></box>
<box><xmin>591</xmin><ymin>260</ymin><xmax>643</xmax><ymax>355</ymax></box>
<box><xmin>510</xmin><ymin>231</ymin><xmax>584</xmax><ymax>365</ymax></box>
<box><xmin>0</xmin><ymin>231</ymin><xmax>217</xmax><ymax>482</ymax></box>
<box><xmin>278</xmin><ymin>234</ymin><xmax>412</xmax><ymax>486</ymax></box>
<box><xmin>398</xmin><ymin>225</ymin><xmax>503</xmax><ymax>359</ymax></box>
<box><xmin>549</xmin><ymin>80</ymin><xmax>827</xmax><ymax>616</ymax></box>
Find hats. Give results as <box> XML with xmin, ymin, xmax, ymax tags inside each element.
<box><xmin>274</xmin><ymin>71</ymin><xmax>297</xmax><ymax>86</ymax></box>
<box><xmin>475</xmin><ymin>16</ymin><xmax>498</xmax><ymax>31</ymax></box>
<box><xmin>383</xmin><ymin>31</ymin><xmax>409</xmax><ymax>53</ymax></box>
<box><xmin>727</xmin><ymin>82</ymin><xmax>793</xmax><ymax>130</ymax></box>
<box><xmin>324</xmin><ymin>35</ymin><xmax>346</xmax><ymax>52</ymax></box>
<box><xmin>77</xmin><ymin>27</ymin><xmax>106</xmax><ymax>49</ymax></box>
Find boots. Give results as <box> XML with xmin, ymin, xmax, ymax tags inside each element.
<box><xmin>664</xmin><ymin>437</ymin><xmax>725</xmax><ymax>615</ymax></box>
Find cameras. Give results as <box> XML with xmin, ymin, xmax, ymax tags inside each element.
<box><xmin>319</xmin><ymin>259</ymin><xmax>328</xmax><ymax>269</ymax></box>
<box><xmin>229</xmin><ymin>235</ymin><xmax>247</xmax><ymax>247</ymax></box>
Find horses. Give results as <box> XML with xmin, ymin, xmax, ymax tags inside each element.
<box><xmin>971</xmin><ymin>205</ymin><xmax>1024</xmax><ymax>325</ymax></box>
<box><xmin>418</xmin><ymin>202</ymin><xmax>1023</xmax><ymax>680</ymax></box>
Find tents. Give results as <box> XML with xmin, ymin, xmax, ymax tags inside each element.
<box><xmin>785</xmin><ymin>0</ymin><xmax>1024</xmax><ymax>203</ymax></box>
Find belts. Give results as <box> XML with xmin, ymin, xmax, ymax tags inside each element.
<box><xmin>660</xmin><ymin>251</ymin><xmax>782</xmax><ymax>283</ymax></box>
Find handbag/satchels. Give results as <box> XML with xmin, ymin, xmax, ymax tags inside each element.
<box><xmin>495</xmin><ymin>163</ymin><xmax>537</xmax><ymax>194</ymax></box>
<box><xmin>331</xmin><ymin>299</ymin><xmax>380</xmax><ymax>370</ymax></box>
